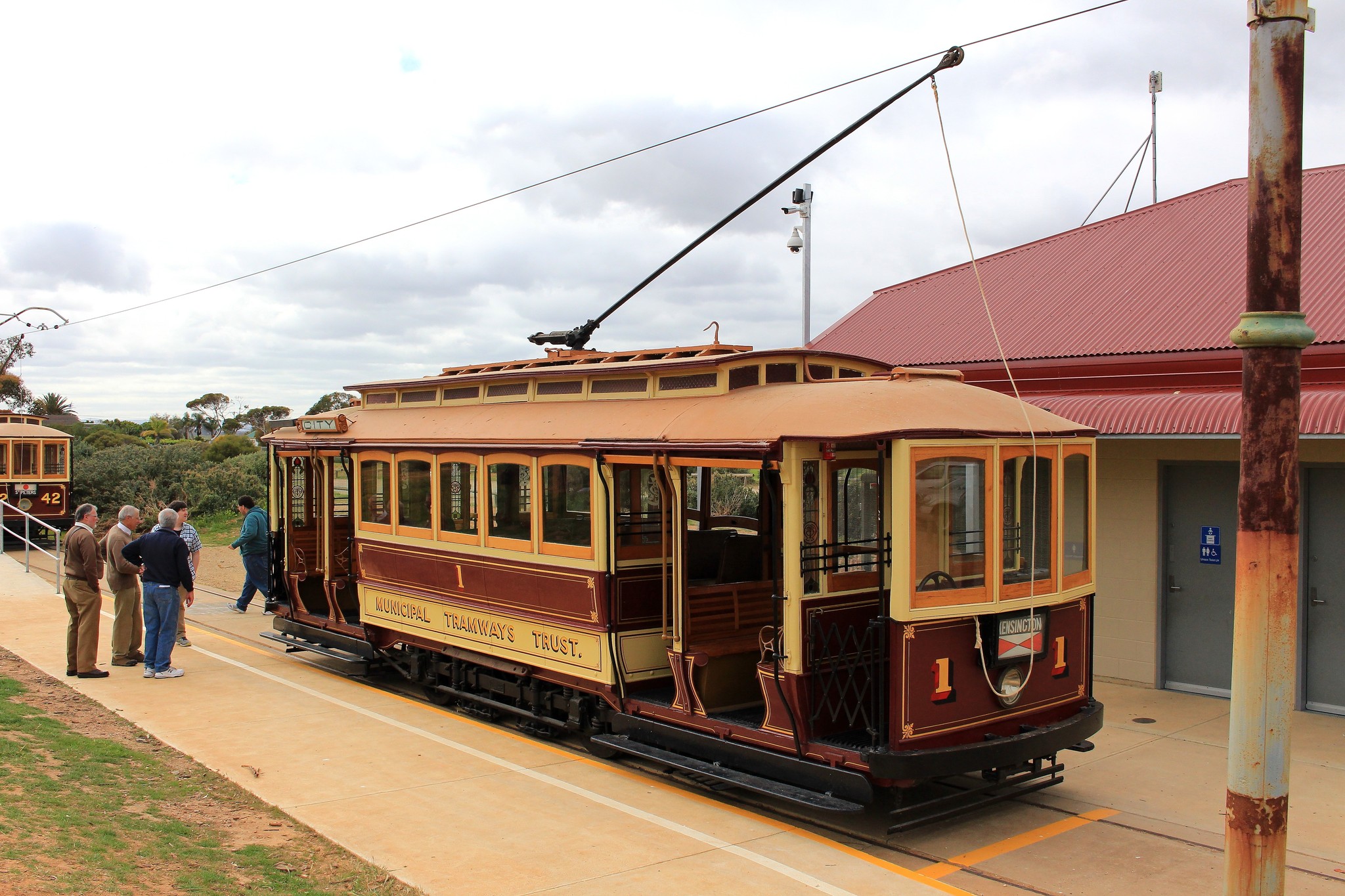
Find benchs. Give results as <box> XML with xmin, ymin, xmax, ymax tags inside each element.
<box><xmin>289</xmin><ymin>526</ymin><xmax>361</xmax><ymax>624</ymax></box>
<box><xmin>665</xmin><ymin>558</ymin><xmax>987</xmax><ymax>740</ymax></box>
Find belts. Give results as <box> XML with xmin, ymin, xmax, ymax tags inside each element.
<box><xmin>65</xmin><ymin>576</ymin><xmax>75</xmax><ymax>580</ymax></box>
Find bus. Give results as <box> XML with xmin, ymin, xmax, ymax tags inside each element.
<box><xmin>259</xmin><ymin>45</ymin><xmax>1105</xmax><ymax>839</ymax></box>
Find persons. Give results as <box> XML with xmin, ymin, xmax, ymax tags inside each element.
<box><xmin>226</xmin><ymin>496</ymin><xmax>278</xmax><ymax>615</ymax></box>
<box><xmin>151</xmin><ymin>501</ymin><xmax>203</xmax><ymax>646</ymax></box>
<box><xmin>63</xmin><ymin>503</ymin><xmax>109</xmax><ymax>679</ymax></box>
<box><xmin>98</xmin><ymin>505</ymin><xmax>145</xmax><ymax>667</ymax></box>
<box><xmin>121</xmin><ymin>509</ymin><xmax>194</xmax><ymax>679</ymax></box>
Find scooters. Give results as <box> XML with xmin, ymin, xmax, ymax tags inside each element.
<box><xmin>0</xmin><ymin>332</ymin><xmax>76</xmax><ymax>547</ymax></box>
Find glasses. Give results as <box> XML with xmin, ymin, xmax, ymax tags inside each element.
<box><xmin>88</xmin><ymin>514</ymin><xmax>98</xmax><ymax>517</ymax></box>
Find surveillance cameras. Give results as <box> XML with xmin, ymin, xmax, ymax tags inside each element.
<box><xmin>787</xmin><ymin>228</ymin><xmax>803</xmax><ymax>253</ymax></box>
<box><xmin>781</xmin><ymin>205</ymin><xmax>807</xmax><ymax>215</ymax></box>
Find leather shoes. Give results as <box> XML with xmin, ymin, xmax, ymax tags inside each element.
<box><xmin>67</xmin><ymin>669</ymin><xmax>78</xmax><ymax>676</ymax></box>
<box><xmin>78</xmin><ymin>668</ymin><xmax>110</xmax><ymax>678</ymax></box>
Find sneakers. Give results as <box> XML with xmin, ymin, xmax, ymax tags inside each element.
<box><xmin>154</xmin><ymin>666</ymin><xmax>184</xmax><ymax>679</ymax></box>
<box><xmin>262</xmin><ymin>611</ymin><xmax>275</xmax><ymax>615</ymax></box>
<box><xmin>143</xmin><ymin>667</ymin><xmax>155</xmax><ymax>677</ymax></box>
<box><xmin>175</xmin><ymin>637</ymin><xmax>191</xmax><ymax>646</ymax></box>
<box><xmin>112</xmin><ymin>656</ymin><xmax>137</xmax><ymax>666</ymax></box>
<box><xmin>128</xmin><ymin>650</ymin><xmax>145</xmax><ymax>662</ymax></box>
<box><xmin>226</xmin><ymin>602</ymin><xmax>245</xmax><ymax>613</ymax></box>
